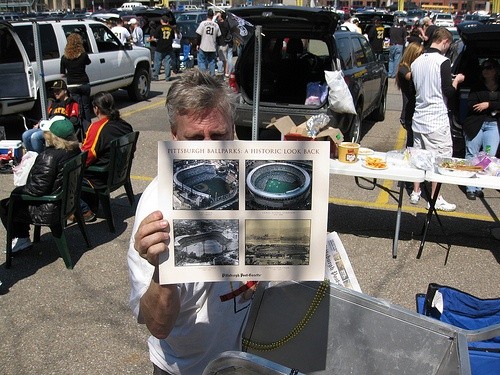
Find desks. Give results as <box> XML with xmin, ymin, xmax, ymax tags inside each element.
<box><xmin>329</xmin><ymin>143</ymin><xmax>500</xmax><ymax>260</ymax></box>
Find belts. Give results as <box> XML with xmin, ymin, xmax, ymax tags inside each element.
<box><xmin>389</xmin><ymin>43</ymin><xmax>402</xmax><ymax>45</ymax></box>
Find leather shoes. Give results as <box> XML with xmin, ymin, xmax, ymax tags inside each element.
<box><xmin>466</xmin><ymin>191</ymin><xmax>475</xmax><ymax>200</ymax></box>
<box><xmin>475</xmin><ymin>191</ymin><xmax>484</xmax><ymax>198</ymax></box>
<box><xmin>69</xmin><ymin>210</ymin><xmax>95</xmax><ymax>221</ymax></box>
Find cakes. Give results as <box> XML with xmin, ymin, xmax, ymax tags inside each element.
<box><xmin>364</xmin><ymin>157</ymin><xmax>387</xmax><ymax>169</ymax></box>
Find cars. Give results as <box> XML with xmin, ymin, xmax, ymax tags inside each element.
<box><xmin>339</xmin><ymin>7</ymin><xmax>500</xmax><ymax>140</ymax></box>
<box><xmin>0</xmin><ymin>2</ymin><xmax>233</xmax><ymax>55</ymax></box>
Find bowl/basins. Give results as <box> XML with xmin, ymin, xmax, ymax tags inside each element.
<box><xmin>338</xmin><ymin>142</ymin><xmax>360</xmax><ymax>163</ymax></box>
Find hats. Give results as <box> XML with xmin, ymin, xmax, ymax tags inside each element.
<box><xmin>40</xmin><ymin>116</ymin><xmax>74</xmax><ymax>141</ymax></box>
<box><xmin>51</xmin><ymin>80</ymin><xmax>67</xmax><ymax>89</ymax></box>
<box><xmin>128</xmin><ymin>18</ymin><xmax>137</xmax><ymax>24</ymax></box>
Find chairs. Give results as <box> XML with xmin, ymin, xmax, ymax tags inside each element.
<box><xmin>6</xmin><ymin>130</ymin><xmax>140</xmax><ymax>271</ymax></box>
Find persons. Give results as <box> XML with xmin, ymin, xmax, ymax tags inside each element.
<box><xmin>411</xmin><ymin>28</ymin><xmax>465</xmax><ymax>212</ymax></box>
<box><xmin>60</xmin><ymin>33</ymin><xmax>93</xmax><ymax>123</ymax></box>
<box><xmin>195</xmin><ymin>10</ymin><xmax>221</xmax><ymax>77</ymax></box>
<box><xmin>0</xmin><ymin>116</ymin><xmax>77</xmax><ymax>255</ymax></box>
<box><xmin>218</xmin><ymin>34</ymin><xmax>233</xmax><ymax>74</ymax></box>
<box><xmin>126</xmin><ymin>72</ymin><xmax>272</xmax><ymax>375</ymax></box>
<box><xmin>212</xmin><ymin>12</ymin><xmax>232</xmax><ymax>77</ymax></box>
<box><xmin>33</xmin><ymin>79</ymin><xmax>79</xmax><ymax>129</ymax></box>
<box><xmin>69</xmin><ymin>93</ymin><xmax>134</xmax><ymax>221</ymax></box>
<box><xmin>396</xmin><ymin>41</ymin><xmax>426</xmax><ymax>189</ymax></box>
<box><xmin>339</xmin><ymin>12</ymin><xmax>437</xmax><ymax>78</ymax></box>
<box><xmin>96</xmin><ymin>16</ymin><xmax>182</xmax><ymax>81</ymax></box>
<box><xmin>460</xmin><ymin>59</ymin><xmax>500</xmax><ymax>200</ymax></box>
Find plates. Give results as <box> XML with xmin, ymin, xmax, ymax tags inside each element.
<box><xmin>359</xmin><ymin>148</ymin><xmax>374</xmax><ymax>154</ymax></box>
<box><xmin>361</xmin><ymin>161</ymin><xmax>387</xmax><ymax>169</ymax></box>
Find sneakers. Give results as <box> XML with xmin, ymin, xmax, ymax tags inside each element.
<box><xmin>225</xmin><ymin>73</ymin><xmax>230</xmax><ymax>77</ymax></box>
<box><xmin>427</xmin><ymin>195</ymin><xmax>456</xmax><ymax>211</ymax></box>
<box><xmin>410</xmin><ymin>190</ymin><xmax>421</xmax><ymax>204</ymax></box>
<box><xmin>4</xmin><ymin>237</ymin><xmax>31</xmax><ymax>254</ymax></box>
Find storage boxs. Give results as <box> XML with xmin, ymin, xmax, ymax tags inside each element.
<box><xmin>266</xmin><ymin>113</ymin><xmax>344</xmax><ymax>148</ymax></box>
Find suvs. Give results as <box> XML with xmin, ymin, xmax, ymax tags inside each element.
<box><xmin>222</xmin><ymin>5</ymin><xmax>389</xmax><ymax>146</ymax></box>
<box><xmin>0</xmin><ymin>16</ymin><xmax>151</xmax><ymax>126</ymax></box>
